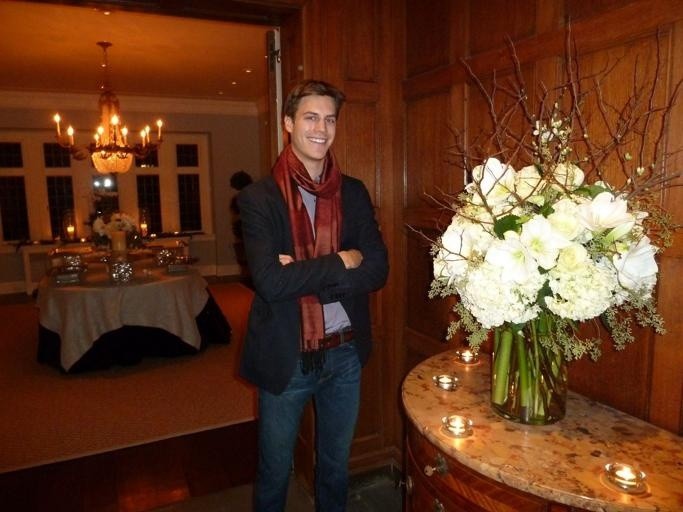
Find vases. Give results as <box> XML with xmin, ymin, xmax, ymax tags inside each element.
<box><xmin>489</xmin><ymin>320</ymin><xmax>571</xmax><ymax>425</ymax></box>
<box><xmin>109</xmin><ymin>232</ymin><xmax>129</xmax><ymax>256</ymax></box>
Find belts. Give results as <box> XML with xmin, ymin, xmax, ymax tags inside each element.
<box><xmin>318</xmin><ymin>326</ymin><xmax>354</xmax><ymax>351</ymax></box>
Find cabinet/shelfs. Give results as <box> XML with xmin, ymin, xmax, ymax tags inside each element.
<box><xmin>399</xmin><ymin>409</ymin><xmax>589</xmax><ymax>512</ymax></box>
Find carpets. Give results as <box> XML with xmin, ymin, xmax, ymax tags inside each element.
<box><xmin>0</xmin><ymin>281</ymin><xmax>260</xmax><ymax>474</ymax></box>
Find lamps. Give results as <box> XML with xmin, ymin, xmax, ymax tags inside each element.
<box><xmin>54</xmin><ymin>41</ymin><xmax>164</xmax><ymax>176</ymax></box>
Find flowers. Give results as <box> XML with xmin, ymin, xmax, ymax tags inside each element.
<box><xmin>92</xmin><ymin>212</ymin><xmax>135</xmax><ymax>237</ymax></box>
<box><xmin>403</xmin><ymin>12</ymin><xmax>682</xmax><ymax>415</ymax></box>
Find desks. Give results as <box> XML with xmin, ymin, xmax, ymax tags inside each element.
<box><xmin>34</xmin><ymin>259</ymin><xmax>232</xmax><ymax>376</ymax></box>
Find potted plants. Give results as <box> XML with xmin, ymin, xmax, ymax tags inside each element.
<box><xmin>229</xmin><ymin>170</ymin><xmax>253</xmax><ymax>278</ymax></box>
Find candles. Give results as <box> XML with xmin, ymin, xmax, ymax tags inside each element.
<box><xmin>449</xmin><ymin>417</ymin><xmax>466</xmax><ymax>434</ymax></box>
<box><xmin>461</xmin><ymin>349</ymin><xmax>473</xmax><ymax>362</ymax></box>
<box><xmin>67</xmin><ymin>225</ymin><xmax>75</xmax><ymax>239</ymax></box>
<box><xmin>140</xmin><ymin>223</ymin><xmax>148</xmax><ymax>237</ymax></box>
<box><xmin>616</xmin><ymin>466</ymin><xmax>635</xmax><ymax>481</ymax></box>
<box><xmin>438</xmin><ymin>376</ymin><xmax>452</xmax><ymax>390</ymax></box>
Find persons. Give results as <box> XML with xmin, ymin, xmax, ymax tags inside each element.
<box><xmin>235</xmin><ymin>78</ymin><xmax>390</xmax><ymax>511</ymax></box>
